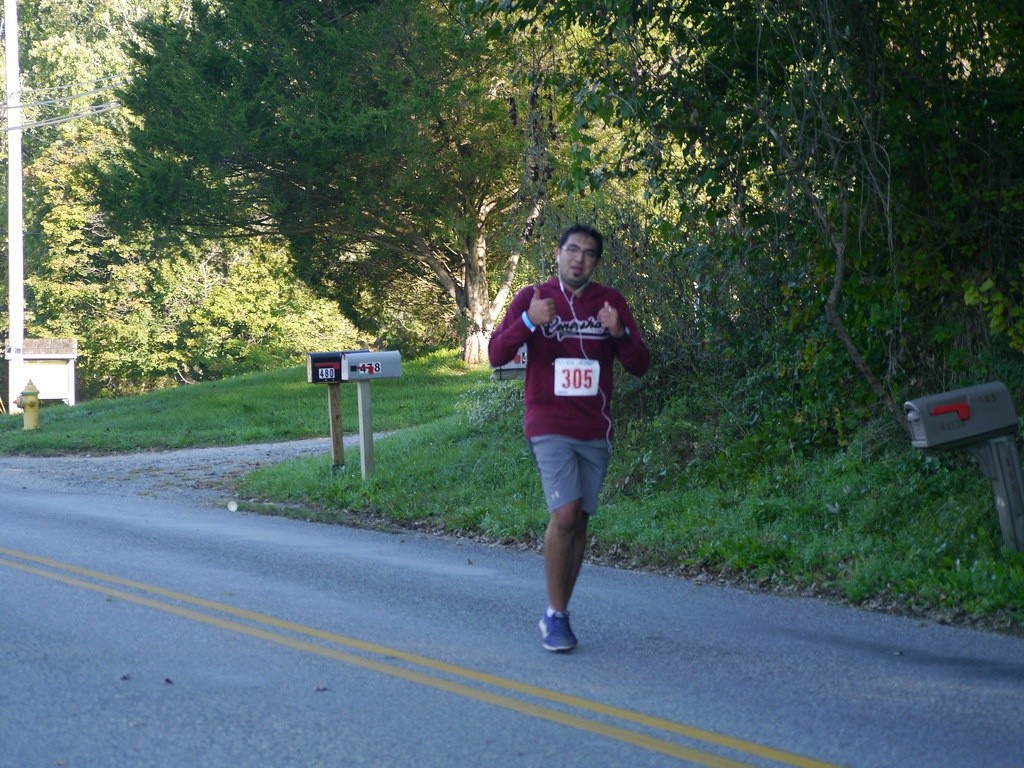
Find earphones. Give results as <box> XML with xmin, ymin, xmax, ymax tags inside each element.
<box><xmin>556</xmin><ymin>255</ymin><xmax>560</xmax><ymax>262</ymax></box>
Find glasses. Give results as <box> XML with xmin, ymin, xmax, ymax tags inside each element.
<box><xmin>560</xmin><ymin>246</ymin><xmax>598</xmax><ymax>264</ymax></box>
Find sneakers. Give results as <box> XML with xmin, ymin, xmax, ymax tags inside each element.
<box><xmin>538</xmin><ymin>608</ymin><xmax>577</xmax><ymax>650</ymax></box>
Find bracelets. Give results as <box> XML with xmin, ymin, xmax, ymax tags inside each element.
<box><xmin>521</xmin><ymin>310</ymin><xmax>537</xmax><ymax>333</ymax></box>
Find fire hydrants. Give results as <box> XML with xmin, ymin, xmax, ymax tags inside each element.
<box><xmin>12</xmin><ymin>379</ymin><xmax>44</xmax><ymax>431</ymax></box>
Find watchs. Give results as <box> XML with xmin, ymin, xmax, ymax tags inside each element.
<box><xmin>611</xmin><ymin>325</ymin><xmax>630</xmax><ymax>341</ymax></box>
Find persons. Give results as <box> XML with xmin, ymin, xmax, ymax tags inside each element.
<box><xmin>489</xmin><ymin>224</ymin><xmax>652</xmax><ymax>652</ymax></box>
